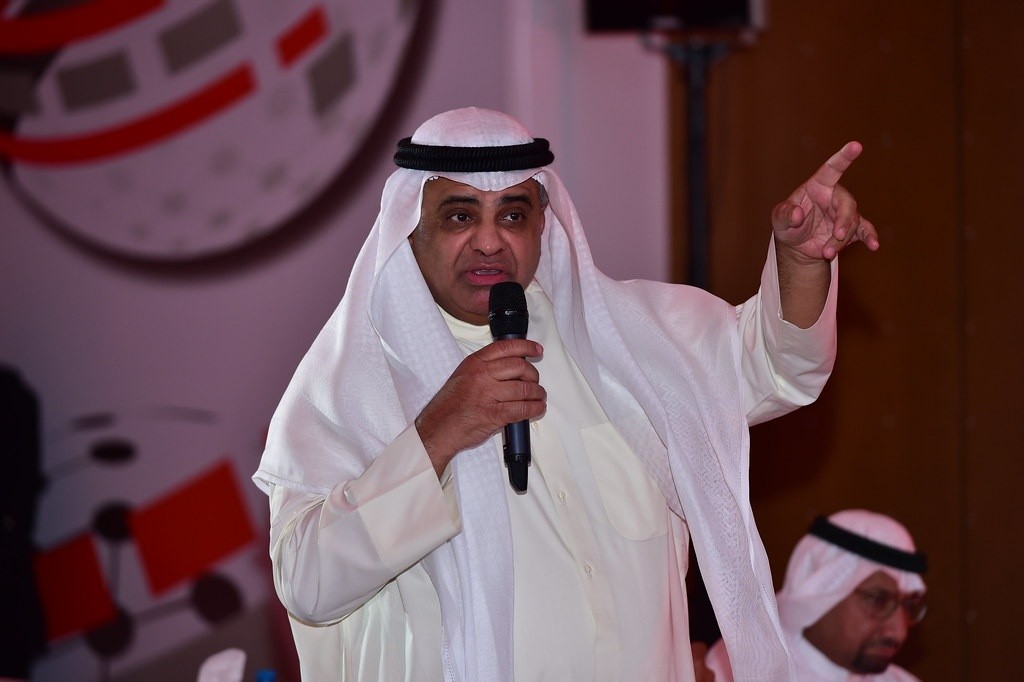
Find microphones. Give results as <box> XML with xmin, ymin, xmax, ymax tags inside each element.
<box><xmin>487</xmin><ymin>281</ymin><xmax>531</xmax><ymax>493</ymax></box>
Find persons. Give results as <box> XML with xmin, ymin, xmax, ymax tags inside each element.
<box><xmin>253</xmin><ymin>106</ymin><xmax>880</xmax><ymax>682</ymax></box>
<box><xmin>0</xmin><ymin>360</ymin><xmax>47</xmax><ymax>682</ymax></box>
<box><xmin>705</xmin><ymin>510</ymin><xmax>929</xmax><ymax>682</ymax></box>
